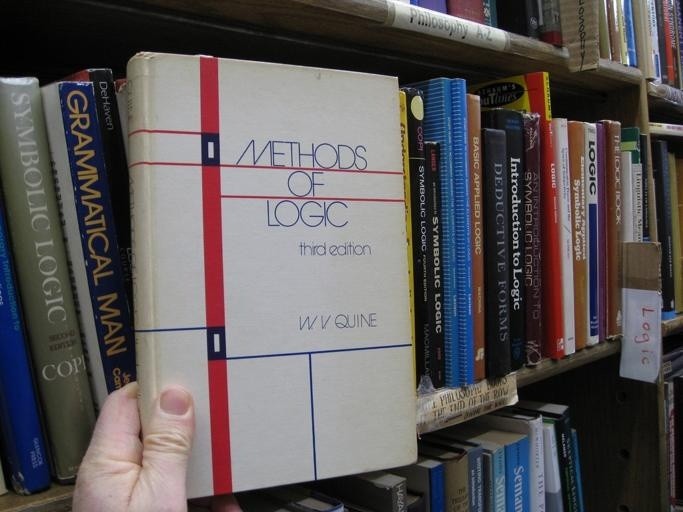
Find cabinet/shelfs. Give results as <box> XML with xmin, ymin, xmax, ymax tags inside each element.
<box><xmin>0</xmin><ymin>0</ymin><xmax>683</xmax><ymax>512</ymax></box>
